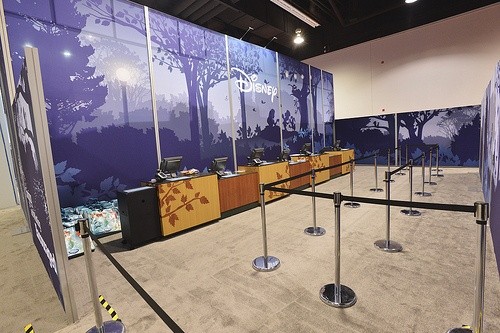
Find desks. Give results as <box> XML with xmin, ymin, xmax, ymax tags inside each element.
<box><xmin>140</xmin><ymin>149</ymin><xmax>356</xmax><ymax>241</ymax></box>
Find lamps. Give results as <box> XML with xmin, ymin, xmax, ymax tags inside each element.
<box><xmin>294</xmin><ymin>28</ymin><xmax>304</xmax><ymax>44</ymax></box>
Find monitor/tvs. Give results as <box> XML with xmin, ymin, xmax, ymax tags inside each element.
<box><xmin>159</xmin><ymin>156</ymin><xmax>182</xmax><ymax>174</ymax></box>
<box><xmin>250</xmin><ymin>148</ymin><xmax>265</xmax><ymax>162</ymax></box>
<box><xmin>279</xmin><ymin>149</ymin><xmax>291</xmax><ymax>160</ymax></box>
<box><xmin>334</xmin><ymin>140</ymin><xmax>341</xmax><ymax>148</ymax></box>
<box><xmin>300</xmin><ymin>143</ymin><xmax>311</xmax><ymax>154</ymax></box>
<box><xmin>210</xmin><ymin>157</ymin><xmax>228</xmax><ymax>173</ymax></box>
<box><xmin>320</xmin><ymin>146</ymin><xmax>331</xmax><ymax>154</ymax></box>
